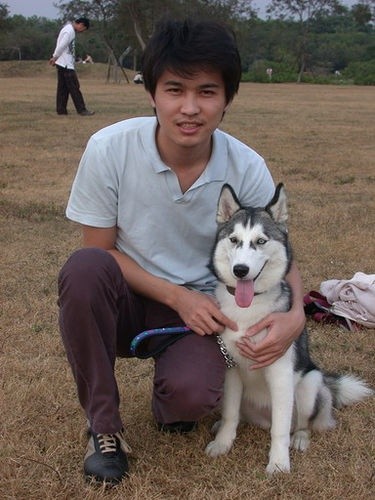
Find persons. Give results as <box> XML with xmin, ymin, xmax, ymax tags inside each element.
<box><xmin>49</xmin><ymin>19</ymin><xmax>95</xmax><ymax>117</ymax></box>
<box><xmin>56</xmin><ymin>19</ymin><xmax>279</xmax><ymax>483</ymax></box>
<box><xmin>133</xmin><ymin>72</ymin><xmax>144</xmax><ymax>84</ymax></box>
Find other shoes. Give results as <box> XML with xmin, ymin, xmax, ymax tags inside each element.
<box><xmin>57</xmin><ymin>111</ymin><xmax>68</xmax><ymax>115</ymax></box>
<box><xmin>80</xmin><ymin>109</ymin><xmax>95</xmax><ymax>115</ymax></box>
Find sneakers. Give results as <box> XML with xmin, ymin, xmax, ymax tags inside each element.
<box><xmin>155</xmin><ymin>421</ymin><xmax>196</xmax><ymax>434</ymax></box>
<box><xmin>83</xmin><ymin>428</ymin><xmax>128</xmax><ymax>487</ymax></box>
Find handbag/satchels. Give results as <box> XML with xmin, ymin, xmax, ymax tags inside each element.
<box><xmin>303</xmin><ymin>272</ymin><xmax>375</xmax><ymax>329</ymax></box>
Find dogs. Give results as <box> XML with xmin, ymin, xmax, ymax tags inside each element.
<box><xmin>206</xmin><ymin>183</ymin><xmax>371</xmax><ymax>477</ymax></box>
